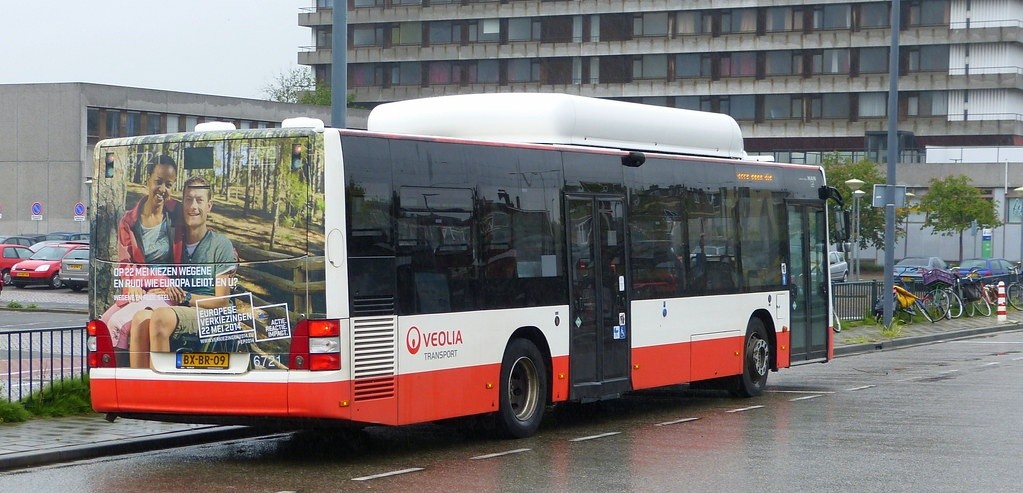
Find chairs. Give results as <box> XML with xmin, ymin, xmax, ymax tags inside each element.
<box><xmin>349</xmin><ymin>228</ymin><xmax>518</xmax><ymax>310</ymax></box>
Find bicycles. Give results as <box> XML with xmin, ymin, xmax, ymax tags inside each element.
<box><xmin>874</xmin><ymin>261</ymin><xmax>1023</xmax><ymax>325</ymax></box>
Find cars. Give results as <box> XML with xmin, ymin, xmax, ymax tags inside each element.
<box><xmin>0</xmin><ymin>231</ymin><xmax>90</xmax><ymax>293</ymax></box>
<box><xmin>957</xmin><ymin>257</ymin><xmax>1023</xmax><ymax>278</ymax></box>
<box><xmin>829</xmin><ymin>249</ymin><xmax>848</xmax><ymax>282</ymax></box>
<box><xmin>893</xmin><ymin>257</ymin><xmax>947</xmax><ymax>279</ymax></box>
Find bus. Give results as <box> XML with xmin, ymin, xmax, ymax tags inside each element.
<box><xmin>84</xmin><ymin>93</ymin><xmax>837</xmax><ymax>436</ymax></box>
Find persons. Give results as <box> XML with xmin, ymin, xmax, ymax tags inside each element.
<box><xmin>128</xmin><ymin>178</ymin><xmax>237</xmax><ymax>368</ymax></box>
<box><xmin>100</xmin><ymin>156</ymin><xmax>187</xmax><ymax>363</ymax></box>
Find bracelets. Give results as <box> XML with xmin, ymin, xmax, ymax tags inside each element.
<box><xmin>184</xmin><ymin>293</ymin><xmax>191</xmax><ymax>305</ymax></box>
